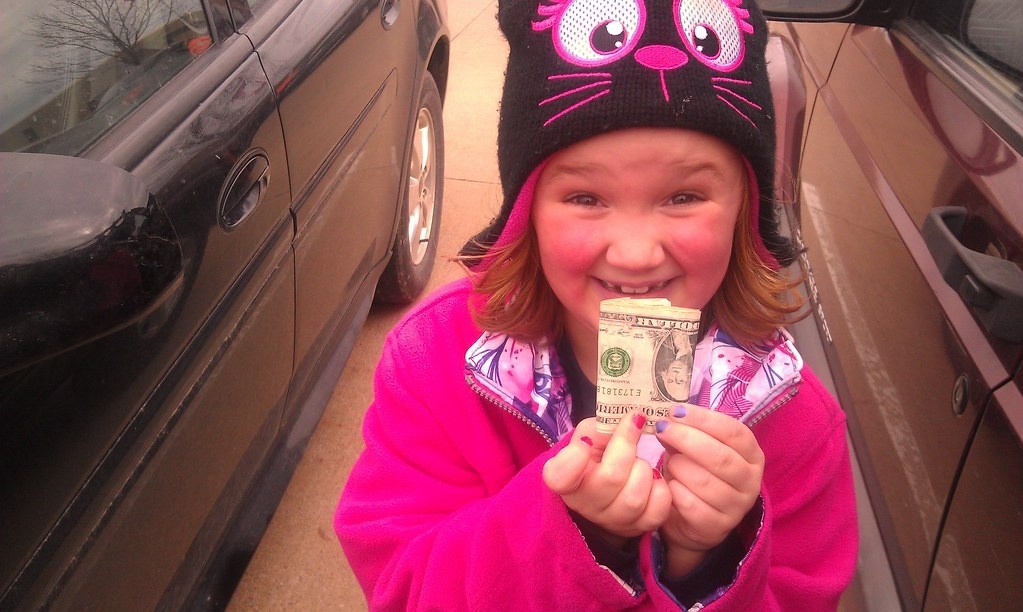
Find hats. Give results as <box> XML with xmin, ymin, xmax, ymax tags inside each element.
<box><xmin>460</xmin><ymin>1</ymin><xmax>803</xmax><ymax>276</ymax></box>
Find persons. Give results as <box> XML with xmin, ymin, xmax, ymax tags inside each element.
<box><xmin>334</xmin><ymin>0</ymin><xmax>858</xmax><ymax>611</ymax></box>
<box><xmin>660</xmin><ymin>330</ymin><xmax>694</xmax><ymax>401</ymax></box>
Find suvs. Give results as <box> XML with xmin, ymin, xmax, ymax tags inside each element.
<box><xmin>746</xmin><ymin>0</ymin><xmax>1023</xmax><ymax>610</ymax></box>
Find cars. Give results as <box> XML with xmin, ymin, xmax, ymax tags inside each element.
<box><xmin>1</xmin><ymin>2</ymin><xmax>451</xmax><ymax>611</ymax></box>
